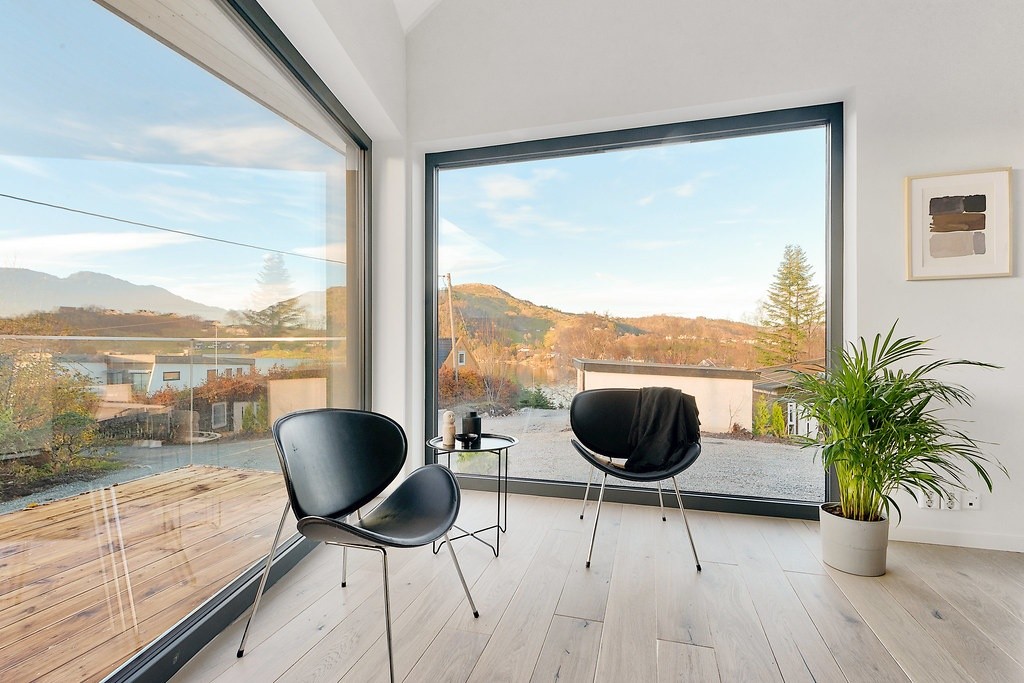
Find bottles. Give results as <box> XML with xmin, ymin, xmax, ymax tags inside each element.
<box><xmin>461</xmin><ymin>412</ymin><xmax>482</xmax><ymax>449</ymax></box>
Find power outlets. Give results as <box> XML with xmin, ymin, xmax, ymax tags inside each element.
<box><xmin>962</xmin><ymin>493</ymin><xmax>981</xmax><ymax>510</ymax></box>
<box><xmin>942</xmin><ymin>490</ymin><xmax>961</xmax><ymax>510</ymax></box>
<box><xmin>918</xmin><ymin>489</ymin><xmax>939</xmax><ymax>510</ymax></box>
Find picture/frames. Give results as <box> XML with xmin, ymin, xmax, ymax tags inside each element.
<box><xmin>903</xmin><ymin>166</ymin><xmax>1015</xmax><ymax>281</ymax></box>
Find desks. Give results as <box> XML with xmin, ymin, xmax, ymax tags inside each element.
<box><xmin>427</xmin><ymin>433</ymin><xmax>519</xmax><ymax>557</ymax></box>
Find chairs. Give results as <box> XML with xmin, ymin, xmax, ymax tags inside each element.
<box><xmin>568</xmin><ymin>387</ymin><xmax>704</xmax><ymax>573</ymax></box>
<box><xmin>236</xmin><ymin>407</ymin><xmax>480</xmax><ymax>683</ymax></box>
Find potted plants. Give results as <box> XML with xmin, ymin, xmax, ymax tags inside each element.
<box><xmin>756</xmin><ymin>316</ymin><xmax>1012</xmax><ymax>577</ymax></box>
